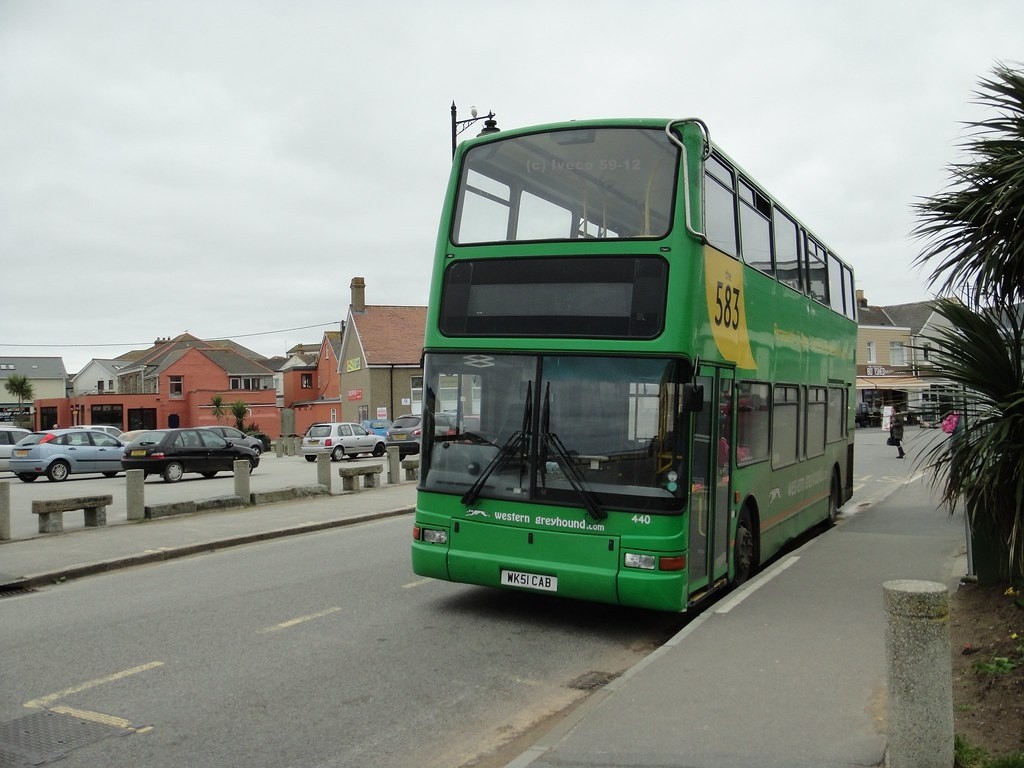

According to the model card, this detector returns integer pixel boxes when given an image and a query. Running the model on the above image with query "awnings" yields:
[856,376,931,393]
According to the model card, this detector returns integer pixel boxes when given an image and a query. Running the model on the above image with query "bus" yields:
[407,114,861,614]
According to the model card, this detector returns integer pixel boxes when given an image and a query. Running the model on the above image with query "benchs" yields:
[33,493,114,533]
[402,459,420,481]
[338,463,384,491]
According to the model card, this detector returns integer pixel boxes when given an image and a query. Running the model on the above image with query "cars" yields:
[57,425,125,445]
[360,419,394,439]
[301,422,387,462]
[10,428,127,483]
[120,428,261,483]
[0,427,34,472]
[177,426,265,456]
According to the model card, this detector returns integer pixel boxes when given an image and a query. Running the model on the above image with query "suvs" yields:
[448,415,481,436]
[386,412,457,462]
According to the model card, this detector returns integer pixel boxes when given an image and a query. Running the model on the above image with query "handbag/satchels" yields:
[887,437,896,446]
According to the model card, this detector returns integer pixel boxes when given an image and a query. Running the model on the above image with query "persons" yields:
[673,397,761,468]
[889,404,906,458]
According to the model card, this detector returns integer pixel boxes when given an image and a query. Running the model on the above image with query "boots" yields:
[896,447,905,458]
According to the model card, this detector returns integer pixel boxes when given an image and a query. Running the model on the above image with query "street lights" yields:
[447,98,504,435]
